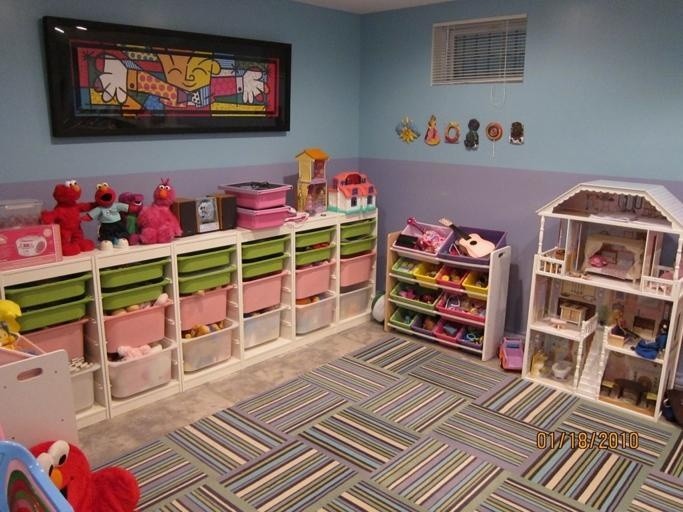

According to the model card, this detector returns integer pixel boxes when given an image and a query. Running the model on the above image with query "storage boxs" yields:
[389,222,507,349]
[216,180,294,230]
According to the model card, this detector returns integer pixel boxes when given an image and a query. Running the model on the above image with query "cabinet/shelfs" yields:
[1,207,379,431]
[385,231,512,362]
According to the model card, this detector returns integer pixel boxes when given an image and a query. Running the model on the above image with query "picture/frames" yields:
[40,15,292,137]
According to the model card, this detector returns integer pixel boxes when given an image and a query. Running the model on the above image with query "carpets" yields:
[89,334,682,511]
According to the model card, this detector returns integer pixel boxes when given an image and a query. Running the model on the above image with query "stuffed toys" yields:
[115,189,142,237]
[0,296,34,355]
[9,438,141,512]
[134,181,184,246]
[79,181,133,249]
[111,283,227,360]
